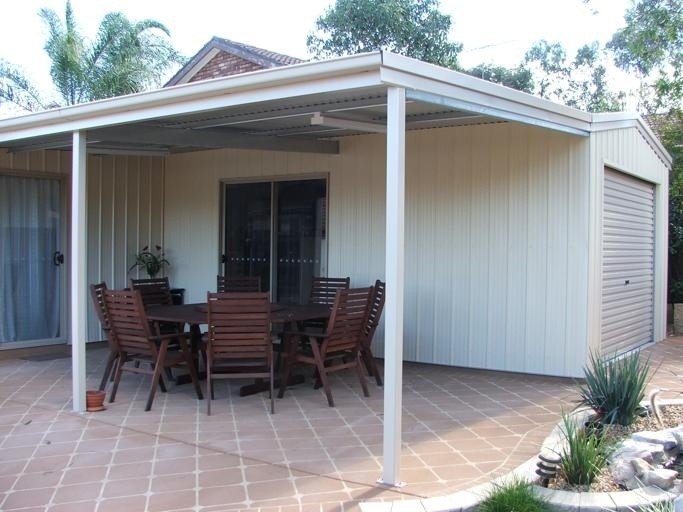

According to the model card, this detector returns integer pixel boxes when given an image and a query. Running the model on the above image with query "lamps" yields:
[310,114,387,135]
[535,450,563,488]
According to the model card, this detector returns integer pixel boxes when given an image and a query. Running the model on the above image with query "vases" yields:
[583,420,604,449]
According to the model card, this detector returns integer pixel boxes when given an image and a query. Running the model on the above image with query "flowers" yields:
[127,244,170,278]
